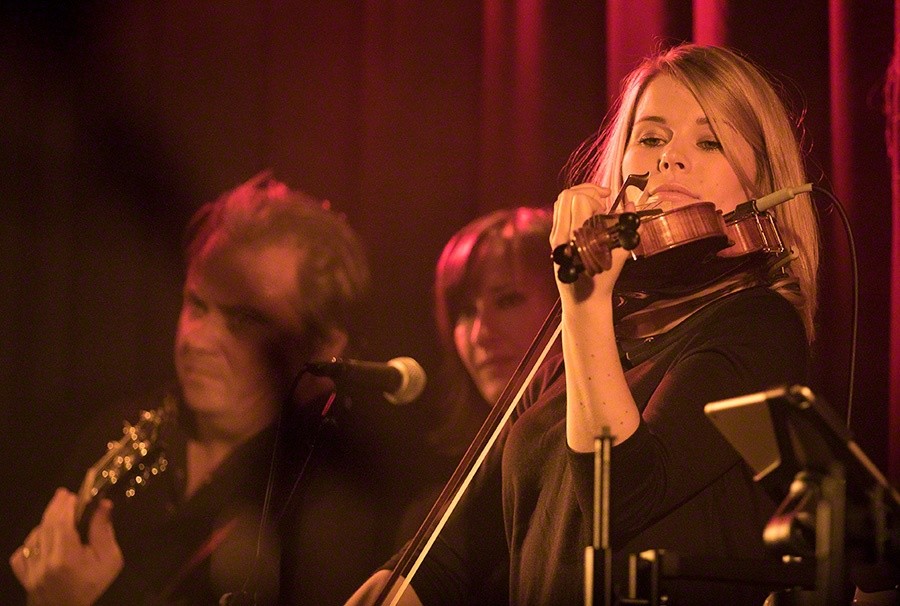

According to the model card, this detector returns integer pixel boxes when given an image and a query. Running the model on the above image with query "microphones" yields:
[306,357,427,405]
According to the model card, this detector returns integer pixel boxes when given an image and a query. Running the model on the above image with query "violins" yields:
[573,198,788,281]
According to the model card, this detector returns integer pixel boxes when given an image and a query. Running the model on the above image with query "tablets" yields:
[707,386,900,594]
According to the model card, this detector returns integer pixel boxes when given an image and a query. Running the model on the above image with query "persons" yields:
[8,43,818,606]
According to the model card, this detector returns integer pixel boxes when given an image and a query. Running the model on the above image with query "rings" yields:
[19,549,43,556]
